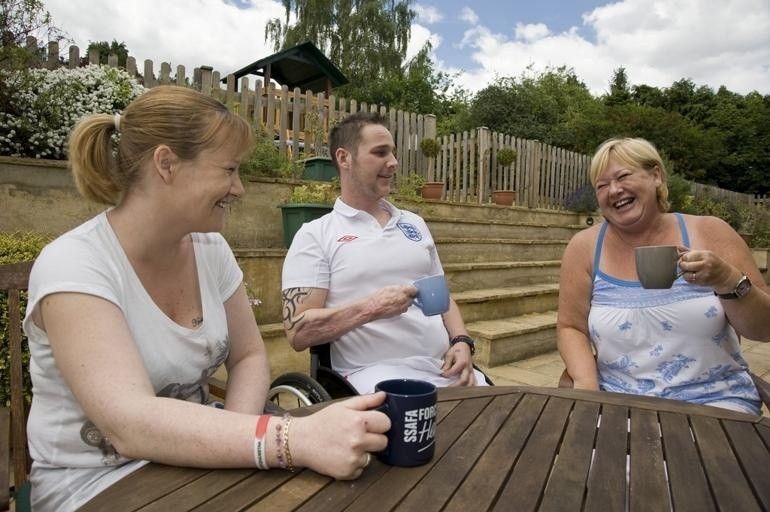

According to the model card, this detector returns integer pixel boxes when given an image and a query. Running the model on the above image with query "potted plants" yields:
[276,179,342,250]
[418,135,444,200]
[492,147,520,206]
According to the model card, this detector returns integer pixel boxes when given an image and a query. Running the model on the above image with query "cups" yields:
[368,378,438,467]
[412,273,450,317]
[635,246,688,288]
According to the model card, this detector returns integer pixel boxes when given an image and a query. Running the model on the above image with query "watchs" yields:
[713,274,752,300]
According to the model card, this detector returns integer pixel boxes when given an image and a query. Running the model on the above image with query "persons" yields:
[280,108,490,404]
[19,85,393,512]
[557,136,770,415]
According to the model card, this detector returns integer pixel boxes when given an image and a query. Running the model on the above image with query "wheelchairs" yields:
[267,344,495,407]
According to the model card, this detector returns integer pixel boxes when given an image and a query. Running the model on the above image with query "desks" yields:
[71,382,769,511]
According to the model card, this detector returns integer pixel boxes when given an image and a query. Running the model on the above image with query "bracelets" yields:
[284,415,293,473]
[274,420,287,470]
[451,333,475,354]
[256,413,270,471]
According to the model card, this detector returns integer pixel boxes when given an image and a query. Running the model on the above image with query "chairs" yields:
[1,261,285,506]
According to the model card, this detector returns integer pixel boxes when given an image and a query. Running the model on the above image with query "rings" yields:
[364,454,373,468]
[692,272,696,282]
